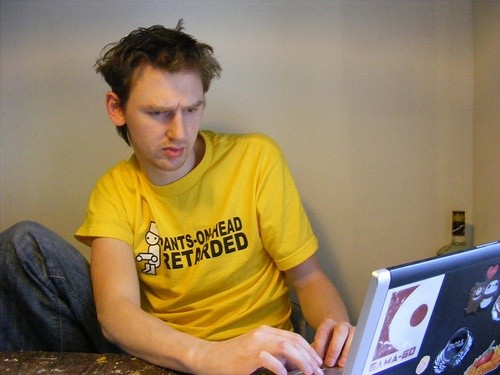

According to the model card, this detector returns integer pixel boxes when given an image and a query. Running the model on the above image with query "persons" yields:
[0,17,359,375]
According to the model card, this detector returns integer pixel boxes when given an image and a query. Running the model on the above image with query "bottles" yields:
[436,209,475,260]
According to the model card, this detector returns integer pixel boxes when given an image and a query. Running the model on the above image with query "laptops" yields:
[272,240,499,375]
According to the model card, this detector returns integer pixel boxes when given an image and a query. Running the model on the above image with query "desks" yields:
[0,350,192,375]
